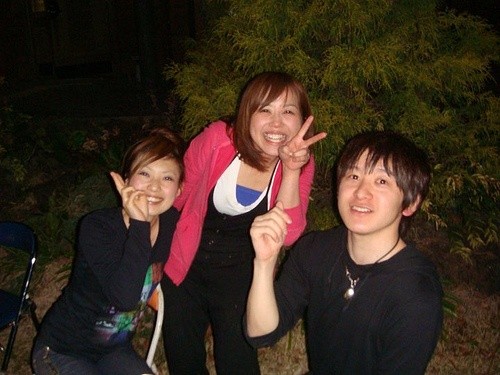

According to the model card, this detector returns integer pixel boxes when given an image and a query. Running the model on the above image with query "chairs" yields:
[0,220,42,374]
[145,281,165,375]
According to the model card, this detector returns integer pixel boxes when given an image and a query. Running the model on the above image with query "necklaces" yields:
[341,234,401,303]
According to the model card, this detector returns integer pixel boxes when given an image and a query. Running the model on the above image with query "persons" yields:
[242,130,446,375]
[160,71,328,375]
[28,127,188,375]
